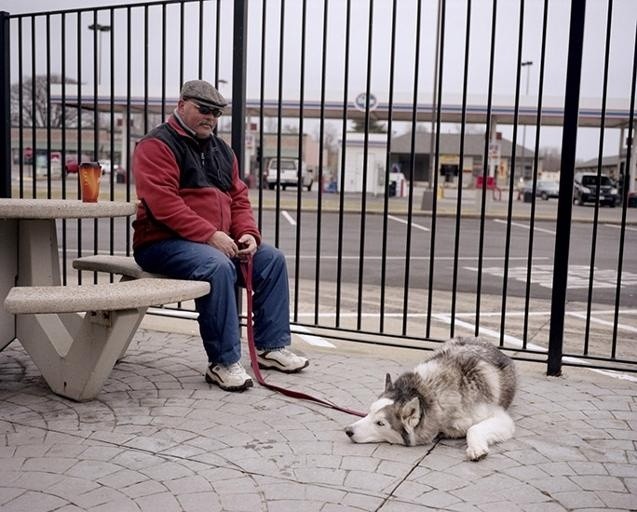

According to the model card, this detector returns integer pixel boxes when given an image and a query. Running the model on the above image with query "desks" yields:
[1,196,140,403]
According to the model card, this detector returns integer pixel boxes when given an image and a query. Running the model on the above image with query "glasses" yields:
[189,101,223,117]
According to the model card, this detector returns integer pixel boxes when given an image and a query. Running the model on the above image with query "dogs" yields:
[345,334,518,463]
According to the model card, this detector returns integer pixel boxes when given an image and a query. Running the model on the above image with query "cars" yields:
[573,171,621,208]
[65,154,111,174]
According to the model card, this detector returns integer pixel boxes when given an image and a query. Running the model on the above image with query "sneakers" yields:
[205,362,254,392]
[251,346,309,373]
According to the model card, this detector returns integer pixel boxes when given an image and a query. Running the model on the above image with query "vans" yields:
[266,157,315,191]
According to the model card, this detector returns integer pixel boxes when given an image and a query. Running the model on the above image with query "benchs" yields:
[2,254,212,404]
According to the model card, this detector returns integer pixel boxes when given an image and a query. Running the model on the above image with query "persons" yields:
[128,78,311,394]
[607,169,622,189]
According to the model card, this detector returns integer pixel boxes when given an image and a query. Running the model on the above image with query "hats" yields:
[181,80,227,110]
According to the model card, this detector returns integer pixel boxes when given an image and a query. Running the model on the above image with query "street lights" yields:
[521,61,533,157]
[88,23,110,86]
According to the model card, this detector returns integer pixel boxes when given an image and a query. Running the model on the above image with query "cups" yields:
[76,159,103,203]
